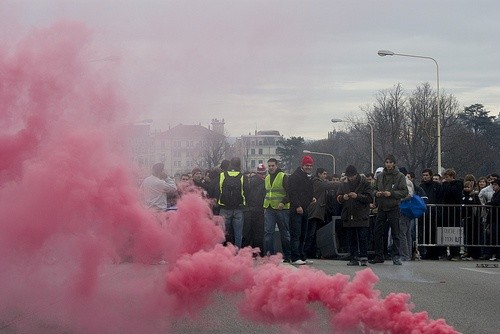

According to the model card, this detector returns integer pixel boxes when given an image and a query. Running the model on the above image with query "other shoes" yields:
[304,259,315,263]
[347,260,359,266]
[292,259,306,265]
[392,257,402,265]
[368,258,383,263]
[420,255,500,261]
[361,260,367,266]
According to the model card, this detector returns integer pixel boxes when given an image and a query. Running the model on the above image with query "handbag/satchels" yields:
[400,194,426,219]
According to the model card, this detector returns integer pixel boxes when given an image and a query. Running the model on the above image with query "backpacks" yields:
[220,172,244,205]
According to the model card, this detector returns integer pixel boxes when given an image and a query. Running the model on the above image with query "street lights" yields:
[332,120,374,175]
[304,150,336,174]
[377,49,443,175]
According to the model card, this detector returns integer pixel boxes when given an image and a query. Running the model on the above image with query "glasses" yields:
[268,165,275,167]
[182,178,188,180]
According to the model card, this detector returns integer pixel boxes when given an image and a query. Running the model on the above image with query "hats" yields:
[256,164,266,173]
[302,156,313,166]
[192,168,201,177]
[345,165,357,176]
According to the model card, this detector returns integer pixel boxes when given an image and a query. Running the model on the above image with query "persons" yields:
[138,155,500,267]
